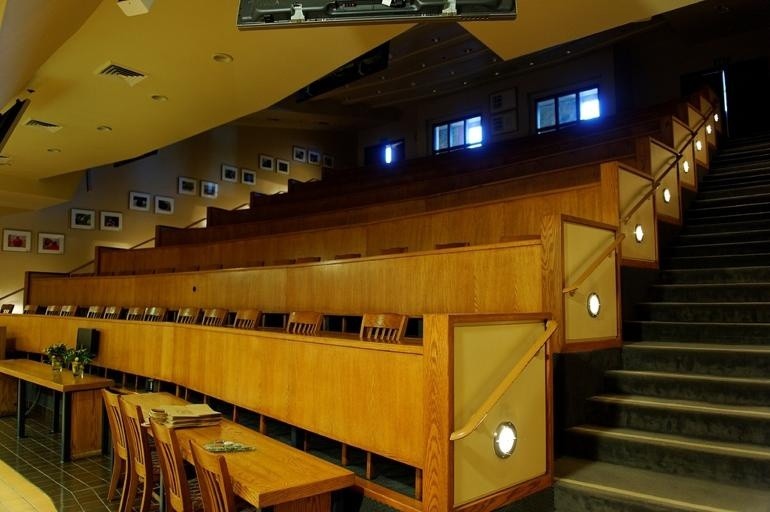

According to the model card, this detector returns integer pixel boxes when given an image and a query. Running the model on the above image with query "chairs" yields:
[1,304,409,510]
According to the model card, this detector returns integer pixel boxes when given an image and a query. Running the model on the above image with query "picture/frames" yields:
[1,228,32,251]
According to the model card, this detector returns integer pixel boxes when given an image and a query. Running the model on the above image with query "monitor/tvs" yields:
[1,97,32,158]
[234,0,519,33]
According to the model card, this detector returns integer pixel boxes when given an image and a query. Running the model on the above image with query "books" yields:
[155,403,224,430]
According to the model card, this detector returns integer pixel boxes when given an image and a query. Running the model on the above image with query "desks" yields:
[23,85,724,353]
